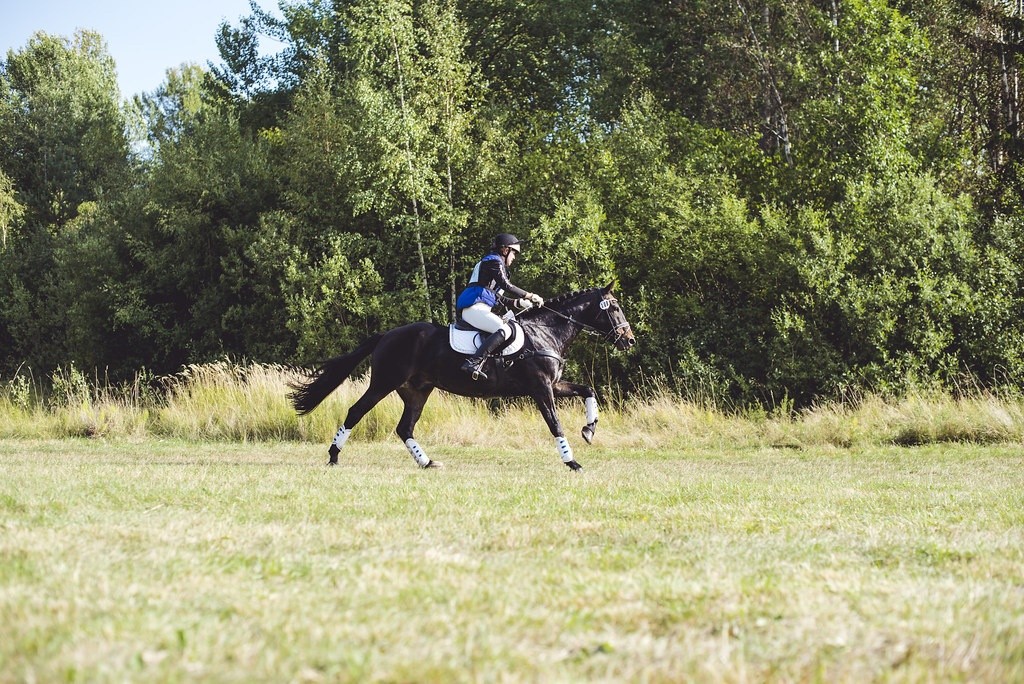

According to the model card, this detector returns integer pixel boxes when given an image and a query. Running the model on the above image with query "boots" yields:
[460,329,506,382]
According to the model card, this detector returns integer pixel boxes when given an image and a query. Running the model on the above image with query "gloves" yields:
[525,292,544,308]
[514,298,533,308]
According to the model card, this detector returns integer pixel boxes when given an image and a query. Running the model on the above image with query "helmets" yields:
[491,234,524,253]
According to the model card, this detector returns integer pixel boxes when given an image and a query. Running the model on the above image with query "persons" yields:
[456,233,544,381]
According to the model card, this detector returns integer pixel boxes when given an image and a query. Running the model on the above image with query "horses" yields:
[284,279,635,472]
